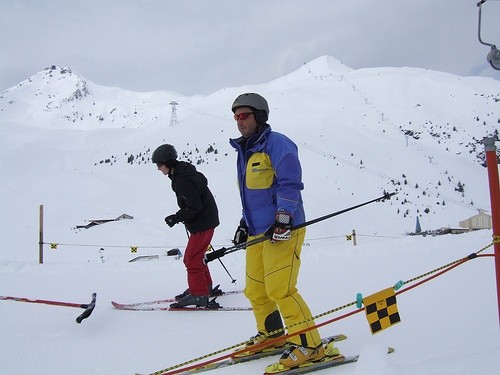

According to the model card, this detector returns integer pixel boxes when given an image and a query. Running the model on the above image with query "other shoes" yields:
[279,341,326,367]
[246,332,286,352]
[178,294,209,308]
[175,290,190,300]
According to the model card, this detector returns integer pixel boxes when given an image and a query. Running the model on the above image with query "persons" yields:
[228,92,326,369]
[149,142,220,310]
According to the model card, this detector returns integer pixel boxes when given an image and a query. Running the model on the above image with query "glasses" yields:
[234,112,254,120]
[157,161,164,170]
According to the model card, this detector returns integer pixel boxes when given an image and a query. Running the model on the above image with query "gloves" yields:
[165,214,178,228]
[233,225,247,249]
[270,212,292,244]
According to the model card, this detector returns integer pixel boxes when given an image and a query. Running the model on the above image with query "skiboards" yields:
[112,289,252,311]
[133,334,359,374]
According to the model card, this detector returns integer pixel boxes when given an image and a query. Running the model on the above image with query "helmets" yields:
[232,93,269,121]
[152,144,177,163]
